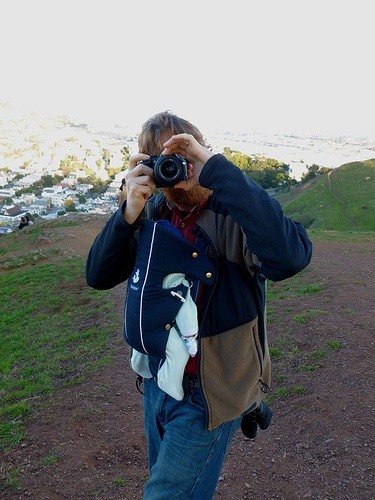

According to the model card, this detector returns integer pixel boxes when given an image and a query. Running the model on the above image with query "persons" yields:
[18,213,34,230]
[85,109,312,500]
[130,219,199,401]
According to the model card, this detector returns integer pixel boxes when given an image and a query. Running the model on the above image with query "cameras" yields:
[141,156,187,188]
